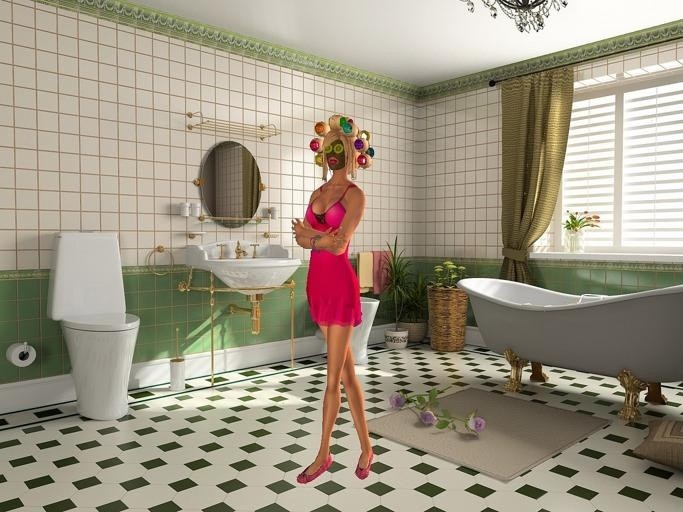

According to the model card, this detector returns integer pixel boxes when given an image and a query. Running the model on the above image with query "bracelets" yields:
[308,237,317,250]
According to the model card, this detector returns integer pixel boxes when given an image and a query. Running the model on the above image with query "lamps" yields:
[459,0,568,34]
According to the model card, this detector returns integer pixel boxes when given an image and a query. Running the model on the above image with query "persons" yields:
[288,112,375,484]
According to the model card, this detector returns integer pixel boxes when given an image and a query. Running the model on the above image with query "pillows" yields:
[633,419,682,473]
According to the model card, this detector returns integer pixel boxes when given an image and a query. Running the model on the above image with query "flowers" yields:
[560,209,602,232]
[389,384,486,435]
[429,261,467,289]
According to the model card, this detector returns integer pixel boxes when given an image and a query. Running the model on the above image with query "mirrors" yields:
[194,141,266,229]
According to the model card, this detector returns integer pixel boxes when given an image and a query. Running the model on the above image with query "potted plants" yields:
[379,234,430,350]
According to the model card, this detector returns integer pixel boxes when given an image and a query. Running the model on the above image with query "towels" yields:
[355,250,392,294]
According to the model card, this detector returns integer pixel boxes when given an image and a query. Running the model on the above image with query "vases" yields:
[427,285,468,352]
[569,232,581,253]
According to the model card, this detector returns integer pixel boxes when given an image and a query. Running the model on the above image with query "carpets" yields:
[352,388,614,482]
